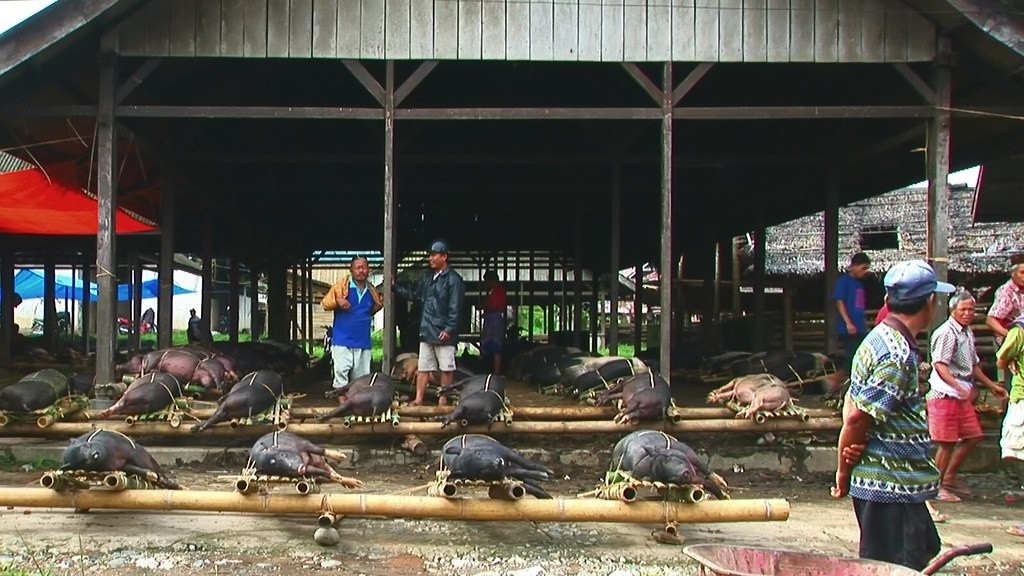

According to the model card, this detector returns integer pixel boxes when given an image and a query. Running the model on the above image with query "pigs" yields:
[0,328,859,503]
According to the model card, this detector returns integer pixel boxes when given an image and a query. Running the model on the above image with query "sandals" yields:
[932,510,947,523]
[1006,526,1024,536]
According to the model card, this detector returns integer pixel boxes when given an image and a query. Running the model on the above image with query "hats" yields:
[1008,254,1024,267]
[429,242,447,254]
[884,260,955,300]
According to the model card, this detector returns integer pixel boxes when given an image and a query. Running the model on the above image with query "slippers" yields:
[936,492,962,503]
[401,400,417,407]
[945,482,974,495]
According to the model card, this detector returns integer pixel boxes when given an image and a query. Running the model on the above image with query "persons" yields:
[142,307,155,334]
[831,260,956,571]
[390,241,464,406]
[824,252,1024,538]
[474,270,507,375]
[189,309,228,342]
[322,256,384,405]
[14,291,22,335]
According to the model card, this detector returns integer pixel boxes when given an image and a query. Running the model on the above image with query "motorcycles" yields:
[30,311,71,334]
[116,308,158,336]
